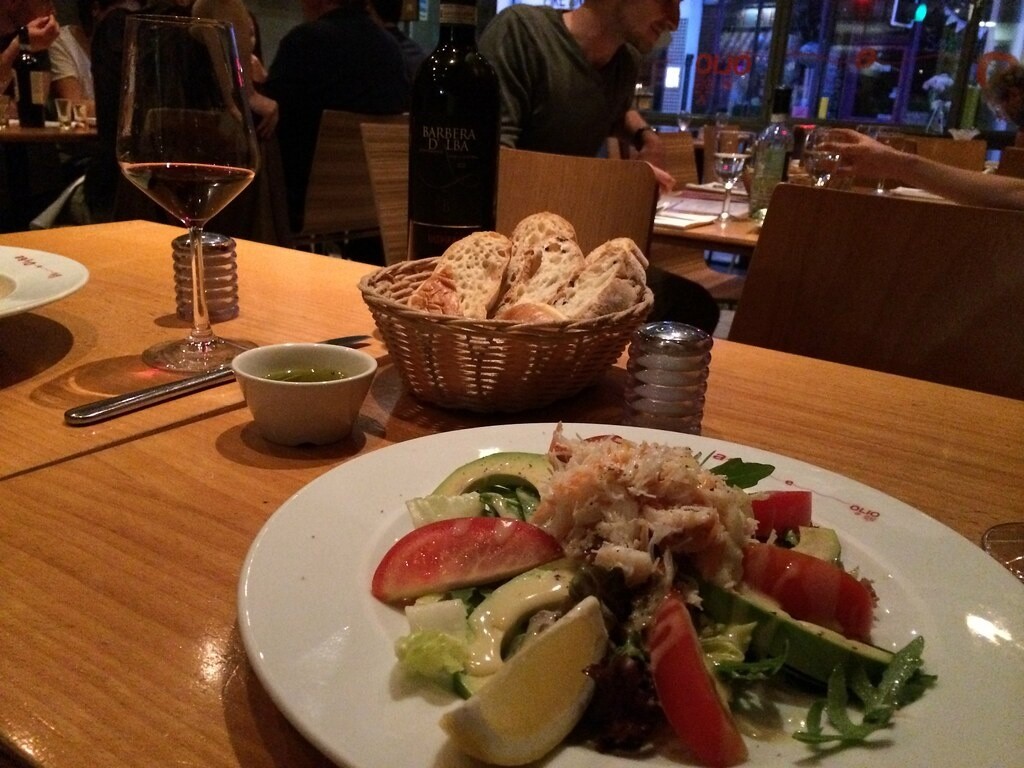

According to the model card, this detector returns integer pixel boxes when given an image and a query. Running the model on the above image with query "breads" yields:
[402,208,648,397]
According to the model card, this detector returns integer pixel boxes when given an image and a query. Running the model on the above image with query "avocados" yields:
[700,579,899,689]
[779,525,841,566]
[451,555,567,701]
[428,451,555,498]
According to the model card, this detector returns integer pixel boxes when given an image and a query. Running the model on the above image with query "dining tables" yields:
[648,167,953,251]
[0,120,99,144]
[0,219,400,485]
[0,335,1024,768]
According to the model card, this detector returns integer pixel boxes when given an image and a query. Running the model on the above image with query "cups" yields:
[800,130,840,186]
[54,98,72,133]
[677,111,691,131]
[73,103,88,131]
[716,112,729,128]
[748,141,784,227]
[233,343,378,446]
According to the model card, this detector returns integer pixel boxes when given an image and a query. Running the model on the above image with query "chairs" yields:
[297,111,1024,404]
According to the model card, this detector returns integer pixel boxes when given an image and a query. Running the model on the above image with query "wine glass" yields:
[116,14,259,374]
[711,129,756,230]
[983,522,1024,586]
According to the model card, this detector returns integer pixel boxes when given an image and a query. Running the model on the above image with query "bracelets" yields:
[634,125,658,152]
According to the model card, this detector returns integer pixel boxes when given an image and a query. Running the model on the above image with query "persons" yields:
[0,0,426,248]
[810,50,1024,212]
[474,0,721,338]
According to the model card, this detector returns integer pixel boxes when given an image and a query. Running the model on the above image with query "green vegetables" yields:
[399,456,937,745]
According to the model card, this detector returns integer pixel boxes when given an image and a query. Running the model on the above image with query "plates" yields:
[653,211,718,229]
[238,423,1024,768]
[0,245,89,317]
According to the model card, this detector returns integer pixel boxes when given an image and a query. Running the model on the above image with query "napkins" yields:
[891,186,947,200]
[651,210,721,230]
[685,181,749,198]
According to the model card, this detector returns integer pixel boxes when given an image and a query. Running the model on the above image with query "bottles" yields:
[172,233,239,322]
[621,322,713,436]
[759,113,793,183]
[11,27,45,129]
[407,0,502,263]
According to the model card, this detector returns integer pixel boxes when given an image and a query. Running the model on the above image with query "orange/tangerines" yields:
[440,596,607,766]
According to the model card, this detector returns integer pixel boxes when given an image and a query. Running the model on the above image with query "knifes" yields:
[63,336,372,425]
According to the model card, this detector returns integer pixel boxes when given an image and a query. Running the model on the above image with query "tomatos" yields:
[647,595,749,766]
[735,540,875,644]
[739,490,816,538]
[373,516,568,600]
